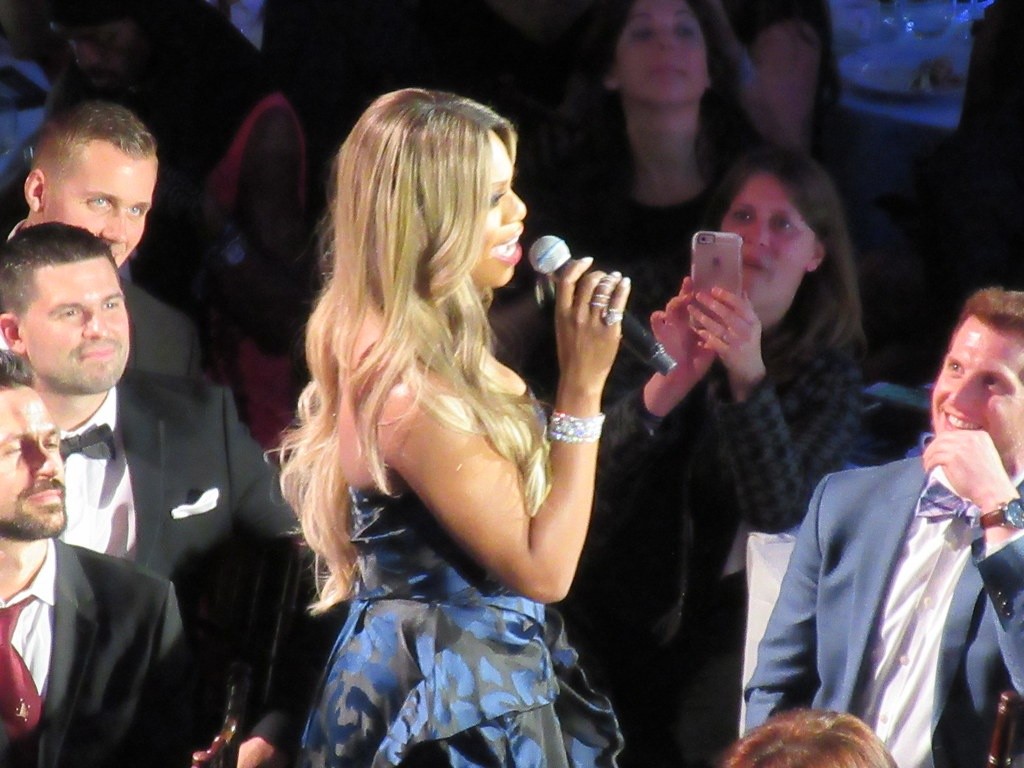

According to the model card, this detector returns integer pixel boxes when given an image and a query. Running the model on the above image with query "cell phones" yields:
[690,231,743,330]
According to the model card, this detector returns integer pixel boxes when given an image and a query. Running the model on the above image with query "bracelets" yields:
[547,408,606,444]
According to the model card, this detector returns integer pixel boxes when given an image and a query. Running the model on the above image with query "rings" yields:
[591,302,609,307]
[593,294,611,299]
[605,308,624,325]
[598,275,618,290]
[721,333,728,342]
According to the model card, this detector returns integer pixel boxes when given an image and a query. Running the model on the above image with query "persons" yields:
[743,284,1024,768]
[0,221,351,768]
[43,1,321,464]
[0,100,204,379]
[592,145,869,768]
[709,706,900,768]
[260,0,1024,249]
[570,1,770,271]
[263,86,626,768]
[0,350,197,768]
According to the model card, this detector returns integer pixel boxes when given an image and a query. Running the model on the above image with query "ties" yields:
[1,594,44,747]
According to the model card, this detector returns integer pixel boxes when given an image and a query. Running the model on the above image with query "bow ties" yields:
[59,423,116,463]
[915,477,981,530]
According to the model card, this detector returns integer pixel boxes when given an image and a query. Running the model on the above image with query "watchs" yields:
[976,497,1024,530]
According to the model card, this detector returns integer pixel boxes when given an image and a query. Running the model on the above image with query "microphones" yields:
[529,235,678,377]
